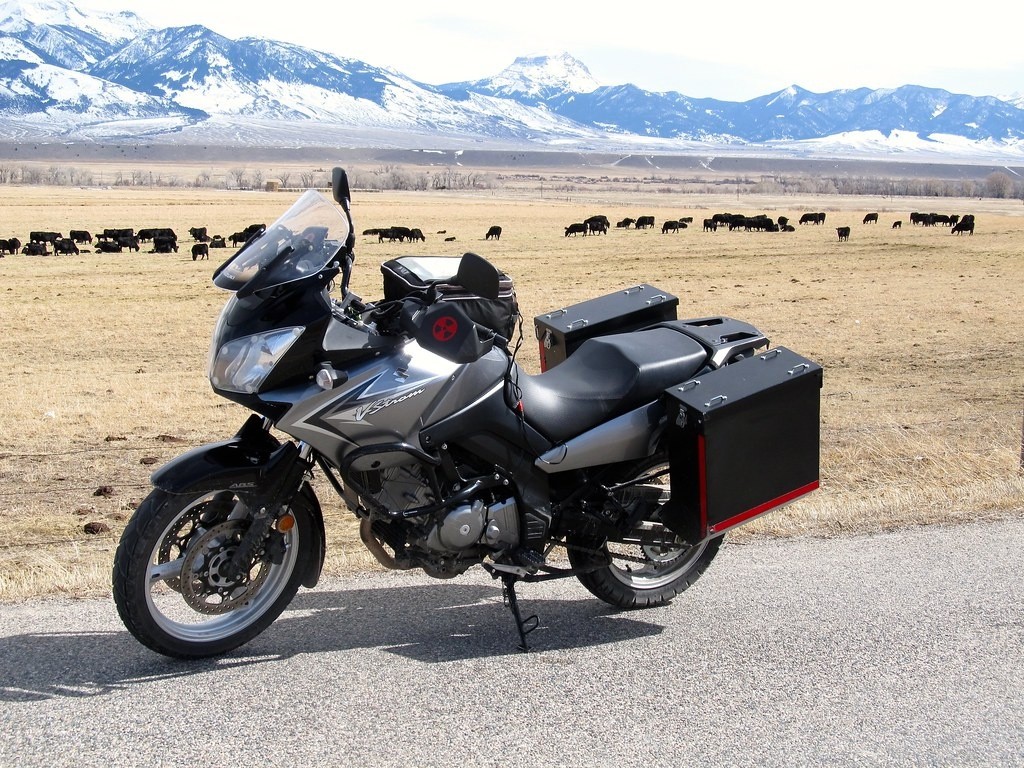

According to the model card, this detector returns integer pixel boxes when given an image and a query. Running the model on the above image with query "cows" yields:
[565,213,795,237]
[363,226,502,243]
[893,221,901,228]
[0,224,266,261]
[836,227,850,242]
[910,212,974,236]
[799,213,826,225]
[863,213,878,224]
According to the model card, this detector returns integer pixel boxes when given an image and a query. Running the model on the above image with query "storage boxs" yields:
[534,283,679,374]
[666,345,823,547]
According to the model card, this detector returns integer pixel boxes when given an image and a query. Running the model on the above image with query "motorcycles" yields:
[109,167,774,660]
